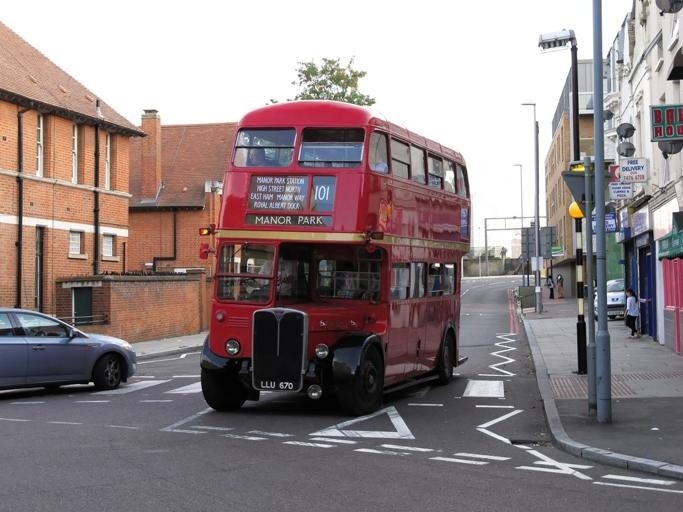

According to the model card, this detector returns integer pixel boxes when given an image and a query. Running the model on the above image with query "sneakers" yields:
[630,330,640,339]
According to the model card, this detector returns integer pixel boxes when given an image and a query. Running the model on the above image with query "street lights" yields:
[538,26,592,374]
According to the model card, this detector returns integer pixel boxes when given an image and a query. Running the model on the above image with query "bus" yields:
[197,99,471,410]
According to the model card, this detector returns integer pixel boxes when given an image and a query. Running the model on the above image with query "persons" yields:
[622,288,639,338]
[371,142,388,173]
[547,275,555,299]
[249,146,281,167]
[255,251,280,286]
[555,272,564,299]
[276,259,305,296]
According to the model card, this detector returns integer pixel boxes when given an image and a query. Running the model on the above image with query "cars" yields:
[593,276,627,320]
[0,307,138,395]
